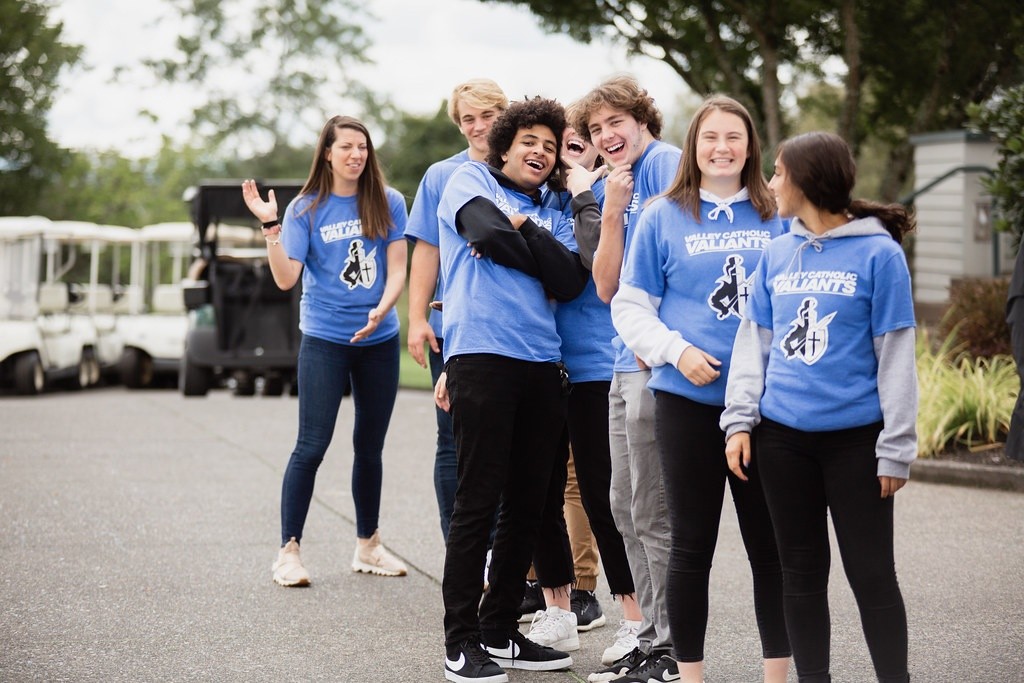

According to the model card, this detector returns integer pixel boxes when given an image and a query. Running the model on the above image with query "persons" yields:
[403,77,683,683]
[610,94,792,683]
[242,115,409,586]
[719,131,919,683]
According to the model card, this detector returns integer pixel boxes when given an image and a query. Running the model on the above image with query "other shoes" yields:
[483,549,492,590]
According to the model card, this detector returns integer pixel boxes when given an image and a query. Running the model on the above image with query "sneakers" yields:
[271,536,311,586]
[352,529,408,576]
[587,647,680,683]
[445,639,508,683]
[570,589,606,631]
[517,579,547,622]
[524,606,580,651]
[602,619,642,666]
[480,629,572,671]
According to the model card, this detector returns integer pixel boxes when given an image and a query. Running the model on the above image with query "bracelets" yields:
[261,217,282,246]
